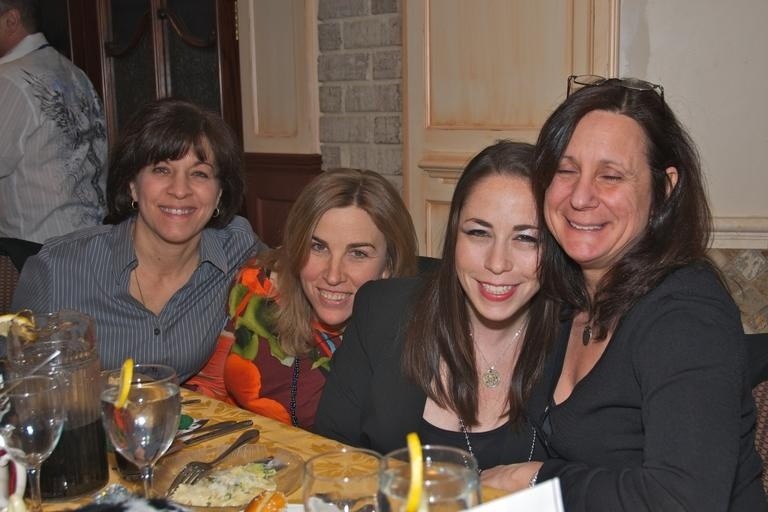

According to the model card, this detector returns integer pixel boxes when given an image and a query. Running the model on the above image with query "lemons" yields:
[114,357,134,409]
[404,432,425,512]
[0,314,36,342]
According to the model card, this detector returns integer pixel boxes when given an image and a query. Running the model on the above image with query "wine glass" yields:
[100,364,182,499]
[0,374,67,511]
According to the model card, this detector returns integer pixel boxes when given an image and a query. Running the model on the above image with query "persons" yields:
[313,139,550,475]
[0,1,113,275]
[468,70,766,511]
[180,165,420,438]
[11,96,275,383]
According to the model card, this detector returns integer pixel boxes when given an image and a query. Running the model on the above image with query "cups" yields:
[6,308,110,504]
[302,444,483,512]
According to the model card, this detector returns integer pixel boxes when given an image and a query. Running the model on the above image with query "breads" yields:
[245,489,289,512]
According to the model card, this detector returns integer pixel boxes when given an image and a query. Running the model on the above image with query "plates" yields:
[152,442,306,512]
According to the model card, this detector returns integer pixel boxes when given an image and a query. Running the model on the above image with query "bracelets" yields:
[525,470,541,490]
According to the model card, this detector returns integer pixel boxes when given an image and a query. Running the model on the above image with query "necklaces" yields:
[453,415,537,470]
[468,317,529,390]
[580,314,599,348]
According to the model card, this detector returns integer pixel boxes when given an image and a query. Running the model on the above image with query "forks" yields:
[168,429,261,496]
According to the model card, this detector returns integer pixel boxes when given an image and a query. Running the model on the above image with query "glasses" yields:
[566,75,666,115]
